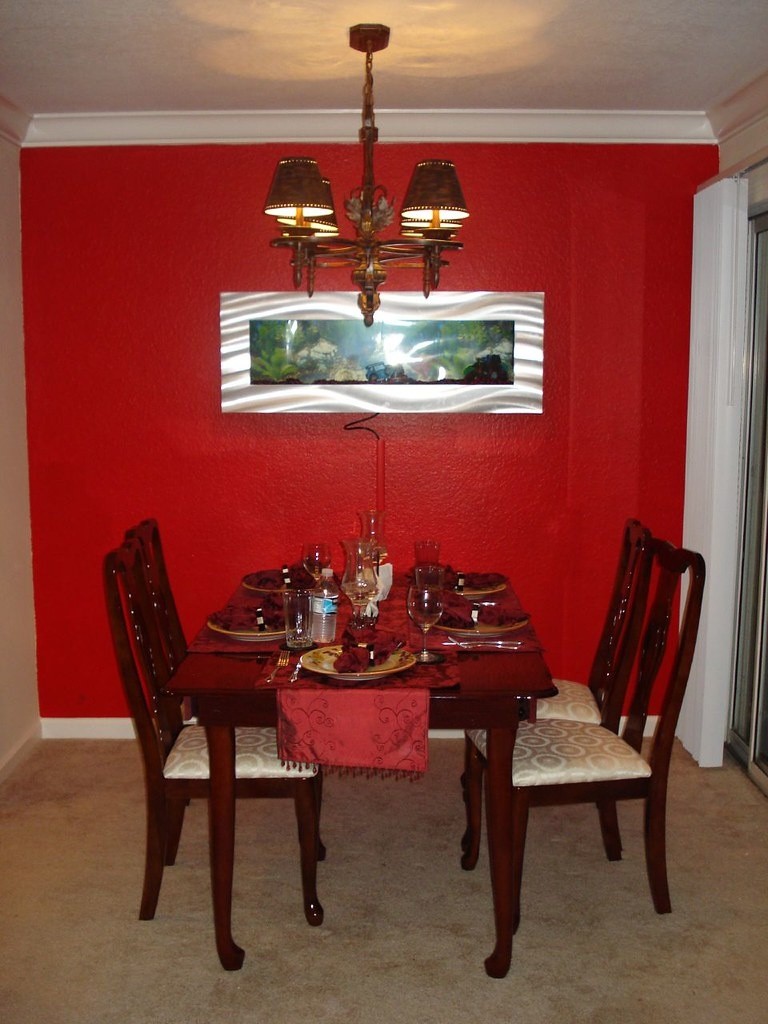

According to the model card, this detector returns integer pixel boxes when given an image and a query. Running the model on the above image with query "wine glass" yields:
[341,538,378,632]
[407,585,444,665]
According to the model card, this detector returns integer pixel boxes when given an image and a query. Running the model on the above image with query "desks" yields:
[165,572,559,979]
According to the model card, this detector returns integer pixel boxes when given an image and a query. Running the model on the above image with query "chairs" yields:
[105,518,326,926]
[462,518,705,934]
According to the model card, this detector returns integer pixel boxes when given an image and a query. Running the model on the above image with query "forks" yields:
[447,635,518,650]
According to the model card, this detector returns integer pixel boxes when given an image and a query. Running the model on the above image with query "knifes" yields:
[442,642,523,646]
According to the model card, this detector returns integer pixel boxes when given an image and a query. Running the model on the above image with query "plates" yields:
[442,580,505,601]
[301,645,415,681]
[207,613,287,643]
[434,608,530,638]
[240,570,314,594]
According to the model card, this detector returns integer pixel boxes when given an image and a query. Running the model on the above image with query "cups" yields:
[413,539,439,568]
[281,590,313,648]
[414,563,442,587]
[303,544,331,586]
[355,508,387,571]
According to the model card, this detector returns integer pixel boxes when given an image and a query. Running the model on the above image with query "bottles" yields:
[309,568,339,643]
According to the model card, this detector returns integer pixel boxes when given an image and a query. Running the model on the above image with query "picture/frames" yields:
[221,291,544,415]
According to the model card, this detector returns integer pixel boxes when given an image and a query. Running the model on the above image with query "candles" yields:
[377,438,385,513]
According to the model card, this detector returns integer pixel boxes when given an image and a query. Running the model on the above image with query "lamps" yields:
[263,24,470,328]
